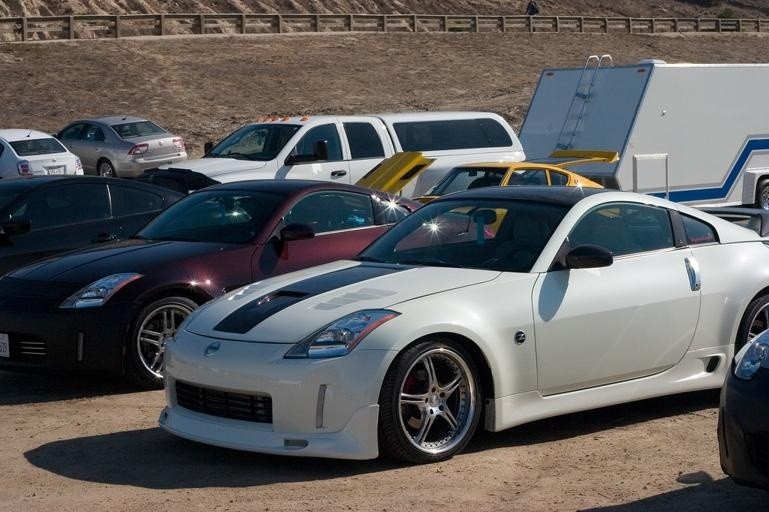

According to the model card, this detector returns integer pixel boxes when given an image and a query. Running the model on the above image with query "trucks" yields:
[519,56,769,210]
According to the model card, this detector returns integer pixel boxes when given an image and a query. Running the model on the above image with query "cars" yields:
[52,117,188,177]
[0,176,185,264]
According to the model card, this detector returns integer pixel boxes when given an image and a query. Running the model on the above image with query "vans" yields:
[0,129,85,177]
[142,112,526,199]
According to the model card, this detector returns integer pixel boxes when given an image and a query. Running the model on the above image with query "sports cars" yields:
[716,326,769,488]
[355,150,620,238]
[160,186,769,465]
[0,178,497,388]
